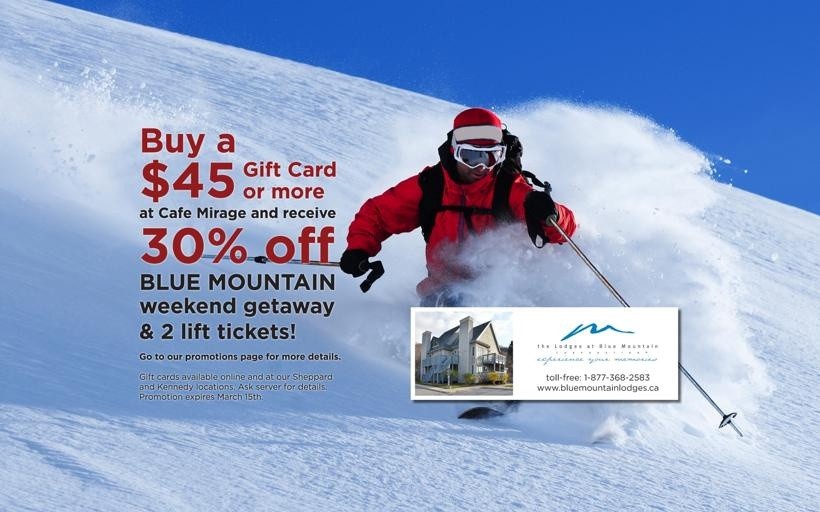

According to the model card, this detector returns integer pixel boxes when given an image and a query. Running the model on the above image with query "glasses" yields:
[453,143,508,169]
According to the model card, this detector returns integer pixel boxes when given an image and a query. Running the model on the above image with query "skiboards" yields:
[456,402,519,418]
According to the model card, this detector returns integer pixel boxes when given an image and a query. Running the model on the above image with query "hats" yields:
[454,109,504,146]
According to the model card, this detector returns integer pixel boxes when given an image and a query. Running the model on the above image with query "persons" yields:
[340,109,579,418]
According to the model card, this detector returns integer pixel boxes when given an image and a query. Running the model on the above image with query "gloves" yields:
[340,248,368,277]
[522,189,558,226]
